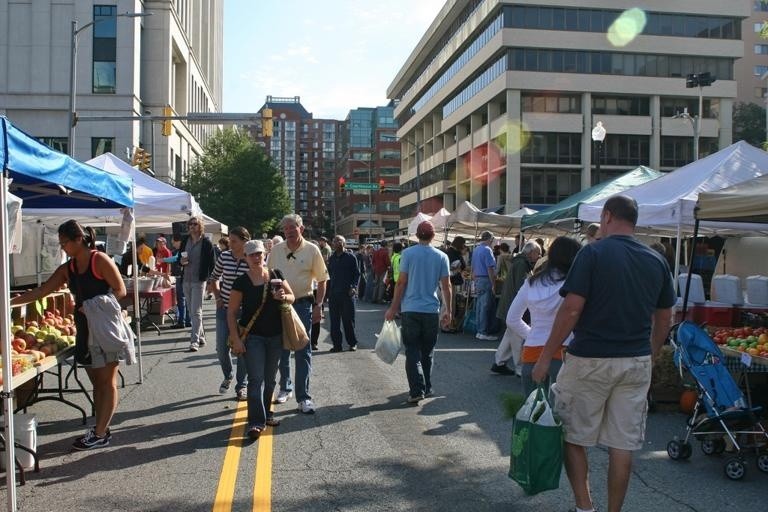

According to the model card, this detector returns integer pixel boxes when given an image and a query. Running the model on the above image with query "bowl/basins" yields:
[123,275,162,292]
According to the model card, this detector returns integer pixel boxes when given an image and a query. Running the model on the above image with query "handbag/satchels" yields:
[280,303,310,350]
[509,414,564,495]
[227,324,248,349]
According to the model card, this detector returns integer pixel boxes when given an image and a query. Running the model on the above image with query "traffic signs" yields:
[346,182,379,190]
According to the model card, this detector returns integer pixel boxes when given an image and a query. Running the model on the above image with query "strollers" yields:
[666,321,768,480]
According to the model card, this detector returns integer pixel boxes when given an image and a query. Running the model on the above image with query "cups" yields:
[271,279,282,295]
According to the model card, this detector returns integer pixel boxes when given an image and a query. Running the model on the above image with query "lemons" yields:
[748,333,768,355]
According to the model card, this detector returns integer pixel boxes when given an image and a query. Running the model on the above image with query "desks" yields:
[457,291,501,332]
[122,284,178,336]
[0,316,132,484]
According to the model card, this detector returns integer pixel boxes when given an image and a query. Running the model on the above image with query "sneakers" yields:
[407,395,425,402]
[275,390,292,403]
[219,376,234,392]
[476,332,499,341]
[491,363,514,375]
[248,427,260,437]
[191,342,199,351]
[266,418,279,425]
[298,400,315,414]
[330,348,342,352]
[200,336,206,345]
[238,387,247,400]
[72,426,112,450]
[350,345,357,351]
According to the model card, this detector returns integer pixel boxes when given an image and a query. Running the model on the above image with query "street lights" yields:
[383,134,422,214]
[348,158,371,235]
[66,9,155,158]
[671,111,699,163]
[591,121,606,184]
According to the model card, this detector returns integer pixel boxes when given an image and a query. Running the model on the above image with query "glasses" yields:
[189,222,198,225]
[59,239,70,246]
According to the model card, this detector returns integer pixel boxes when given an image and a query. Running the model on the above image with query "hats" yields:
[588,223,602,239]
[243,240,265,256]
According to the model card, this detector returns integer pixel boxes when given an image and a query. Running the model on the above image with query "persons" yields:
[385,220,452,402]
[227,240,296,435]
[532,195,678,512]
[11,221,127,453]
[123,213,725,435]
[209,227,252,399]
[266,214,331,414]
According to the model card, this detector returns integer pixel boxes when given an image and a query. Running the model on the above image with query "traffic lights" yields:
[339,178,345,193]
[380,181,385,192]
[131,147,144,168]
[140,152,152,170]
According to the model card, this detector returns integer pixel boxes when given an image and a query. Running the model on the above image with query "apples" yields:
[713,326,768,348]
[11,309,76,362]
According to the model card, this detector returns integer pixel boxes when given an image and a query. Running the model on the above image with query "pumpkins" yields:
[680,390,698,412]
